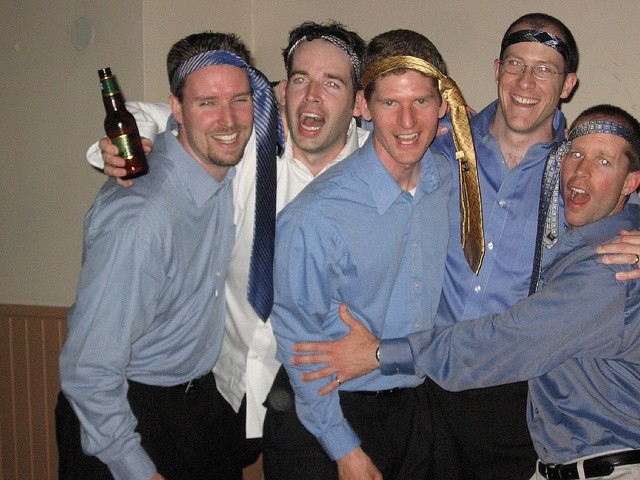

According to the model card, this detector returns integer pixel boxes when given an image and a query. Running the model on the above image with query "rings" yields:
[631,254,639,266]
[336,377,341,385]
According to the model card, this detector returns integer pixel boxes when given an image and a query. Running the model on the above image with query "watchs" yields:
[376,347,380,361]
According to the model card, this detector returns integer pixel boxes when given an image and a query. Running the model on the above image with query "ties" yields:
[361,56,487,274]
[285,32,361,85]
[528,118,638,297]
[172,49,285,323]
[502,28,570,77]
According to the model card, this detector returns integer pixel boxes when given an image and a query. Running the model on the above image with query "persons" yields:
[261,27,454,480]
[428,12,640,480]
[53,29,252,480]
[86,18,449,480]
[291,102,640,480]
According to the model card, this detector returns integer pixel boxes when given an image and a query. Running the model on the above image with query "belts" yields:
[536,451,640,480]
